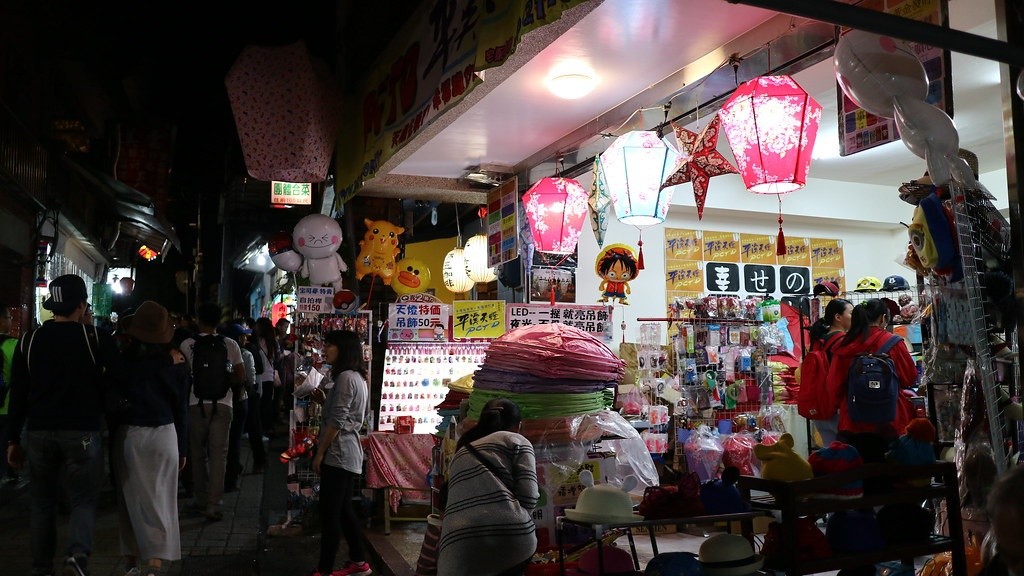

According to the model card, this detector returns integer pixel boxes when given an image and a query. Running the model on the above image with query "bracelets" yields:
[7,440,19,445]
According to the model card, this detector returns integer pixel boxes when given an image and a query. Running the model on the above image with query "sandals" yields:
[200,509,224,521]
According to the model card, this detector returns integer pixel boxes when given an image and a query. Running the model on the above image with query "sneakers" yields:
[64,557,85,576]
[329,560,372,576]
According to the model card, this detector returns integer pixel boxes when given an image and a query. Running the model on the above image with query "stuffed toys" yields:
[905,202,958,277]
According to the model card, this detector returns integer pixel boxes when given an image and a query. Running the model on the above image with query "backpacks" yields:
[798,332,848,420]
[191,334,230,400]
[847,335,904,422]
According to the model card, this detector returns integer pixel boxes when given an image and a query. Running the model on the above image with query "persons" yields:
[792,298,917,452]
[436,398,541,576]
[307,329,372,576]
[0,303,295,520]
[104,302,193,576]
[9,273,116,576]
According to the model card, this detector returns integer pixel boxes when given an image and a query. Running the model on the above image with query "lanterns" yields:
[224,29,335,184]
[598,131,678,275]
[715,72,822,257]
[523,174,588,309]
[442,231,499,302]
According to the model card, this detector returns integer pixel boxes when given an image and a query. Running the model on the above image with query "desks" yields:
[368,430,437,538]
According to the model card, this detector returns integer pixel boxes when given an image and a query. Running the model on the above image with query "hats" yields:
[639,486,681,514]
[754,418,936,559]
[121,301,174,343]
[854,276,883,291]
[228,324,252,334]
[879,275,910,291]
[700,482,752,514]
[646,552,704,576]
[42,274,88,310]
[564,470,645,522]
[700,534,764,576]
[575,546,642,576]
[814,282,839,296]
